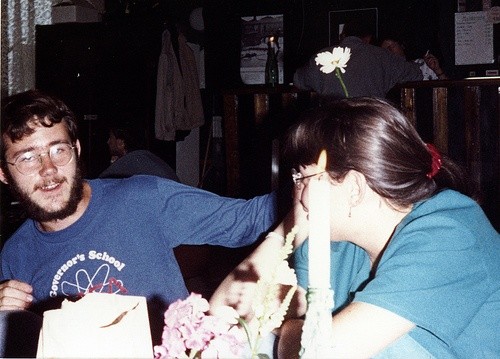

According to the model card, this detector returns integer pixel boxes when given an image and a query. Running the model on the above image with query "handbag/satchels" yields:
[30,291,155,357]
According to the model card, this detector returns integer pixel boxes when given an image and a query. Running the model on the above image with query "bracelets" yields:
[266,232,297,252]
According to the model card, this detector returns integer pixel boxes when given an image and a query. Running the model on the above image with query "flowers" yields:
[152,223,300,359]
[315,46,354,98]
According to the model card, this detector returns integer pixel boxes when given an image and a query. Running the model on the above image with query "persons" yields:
[0,90,297,359]
[292,15,422,110]
[207,96,500,359]
[379,37,408,62]
[95,118,177,182]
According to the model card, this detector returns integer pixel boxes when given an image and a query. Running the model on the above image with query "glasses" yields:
[289,166,331,189]
[2,144,79,175]
